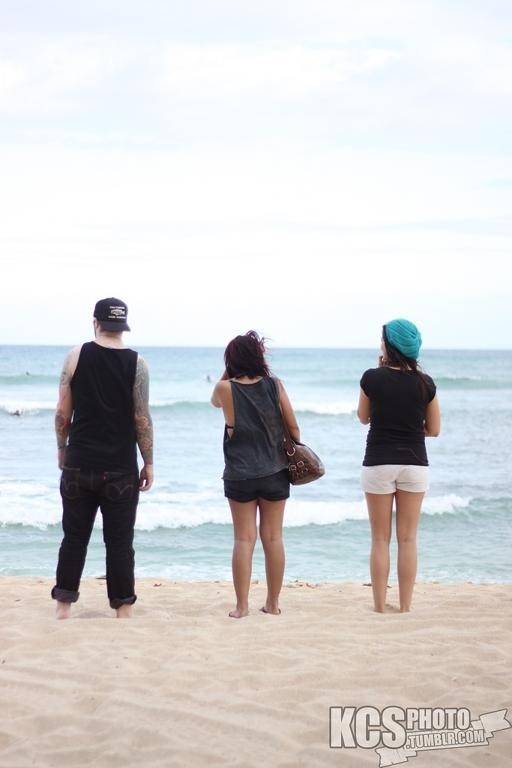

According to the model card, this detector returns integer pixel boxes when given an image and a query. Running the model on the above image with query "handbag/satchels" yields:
[284,440,325,485]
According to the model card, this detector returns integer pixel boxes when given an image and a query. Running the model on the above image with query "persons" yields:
[357,316,440,613]
[50,297,153,620]
[211,327,301,618]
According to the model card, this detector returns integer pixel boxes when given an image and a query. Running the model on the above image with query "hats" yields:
[94,297,131,332]
[385,319,422,361]
